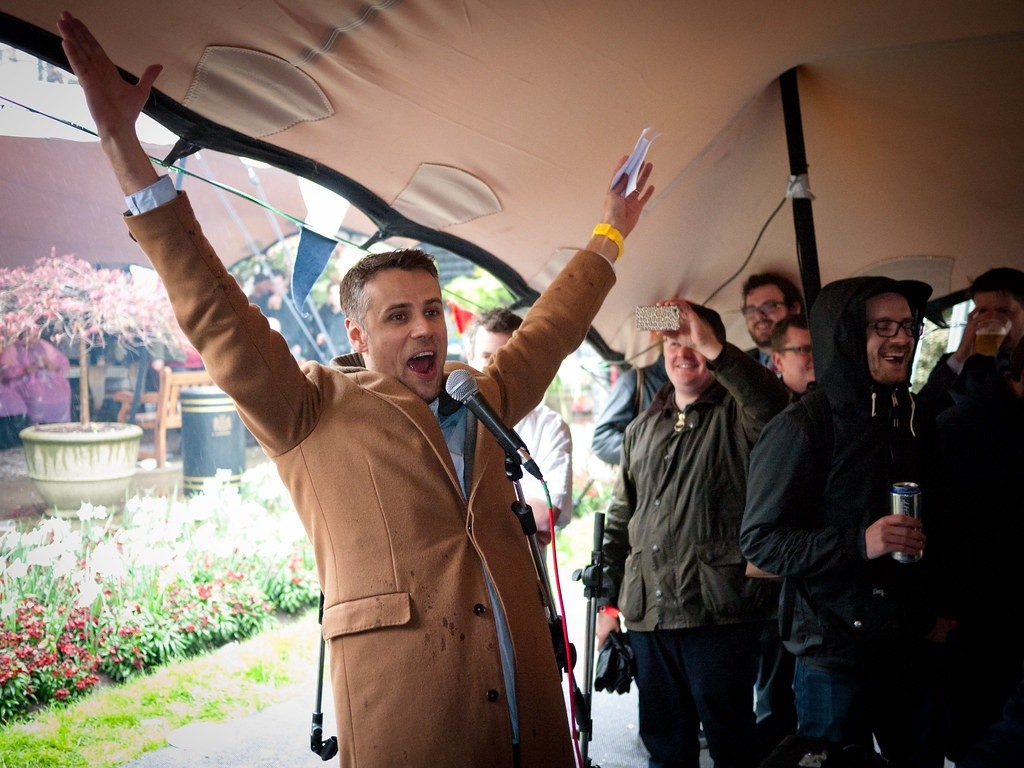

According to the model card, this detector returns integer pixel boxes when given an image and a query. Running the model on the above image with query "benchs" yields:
[113,369,215,470]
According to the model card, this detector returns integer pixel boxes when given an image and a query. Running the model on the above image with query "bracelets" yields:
[598,605,619,618]
[592,223,625,260]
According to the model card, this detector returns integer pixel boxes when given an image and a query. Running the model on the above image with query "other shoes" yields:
[698,728,709,750]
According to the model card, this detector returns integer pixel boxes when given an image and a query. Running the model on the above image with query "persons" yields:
[151,304,205,372]
[739,267,1024,768]
[741,272,805,370]
[465,308,573,551]
[769,313,816,402]
[596,298,791,768]
[0,322,71,451]
[590,351,669,466]
[57,11,655,768]
[249,269,351,365]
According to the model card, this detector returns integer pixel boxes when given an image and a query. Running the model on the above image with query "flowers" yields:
[0,246,195,429]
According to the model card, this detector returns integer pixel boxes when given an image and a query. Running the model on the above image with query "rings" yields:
[635,190,640,193]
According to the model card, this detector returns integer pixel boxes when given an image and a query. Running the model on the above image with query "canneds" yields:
[889,481,925,564]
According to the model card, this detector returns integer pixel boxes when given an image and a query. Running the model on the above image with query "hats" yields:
[864,280,934,312]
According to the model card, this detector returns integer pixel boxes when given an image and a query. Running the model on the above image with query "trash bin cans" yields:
[179,386,246,496]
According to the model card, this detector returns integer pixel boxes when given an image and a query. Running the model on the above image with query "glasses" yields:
[868,321,925,338]
[778,344,813,357]
[742,300,791,320]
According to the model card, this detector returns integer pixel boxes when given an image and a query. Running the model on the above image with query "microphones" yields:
[446,370,543,479]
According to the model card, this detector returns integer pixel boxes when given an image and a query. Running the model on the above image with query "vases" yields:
[18,421,143,518]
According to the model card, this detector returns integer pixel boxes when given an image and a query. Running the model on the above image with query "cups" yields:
[973,312,1012,356]
